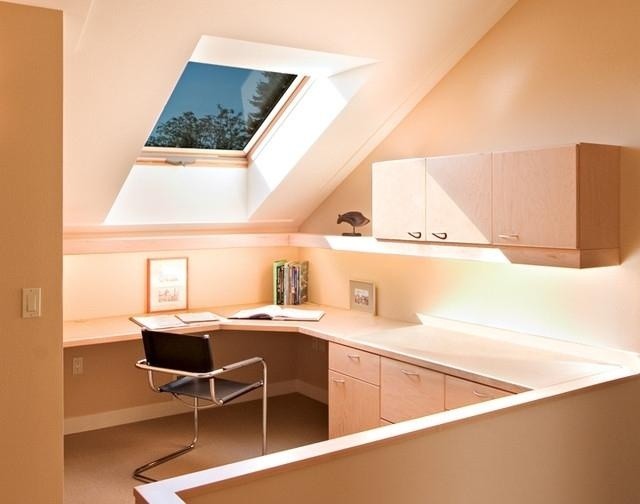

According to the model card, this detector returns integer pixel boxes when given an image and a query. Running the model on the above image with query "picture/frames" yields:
[347,278,376,316]
[144,255,191,314]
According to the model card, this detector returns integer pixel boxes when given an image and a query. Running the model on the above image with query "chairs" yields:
[131,325,269,487]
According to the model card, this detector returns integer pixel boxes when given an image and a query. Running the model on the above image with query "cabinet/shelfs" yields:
[322,339,381,441]
[366,152,491,248]
[133,356,640,501]
[486,138,623,269]
[444,366,518,412]
[378,351,444,426]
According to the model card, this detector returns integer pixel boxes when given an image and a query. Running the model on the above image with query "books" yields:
[228,305,325,323]
[274,258,310,306]
[129,310,222,329]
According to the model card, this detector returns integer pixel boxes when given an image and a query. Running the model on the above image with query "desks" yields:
[64,301,409,355]
